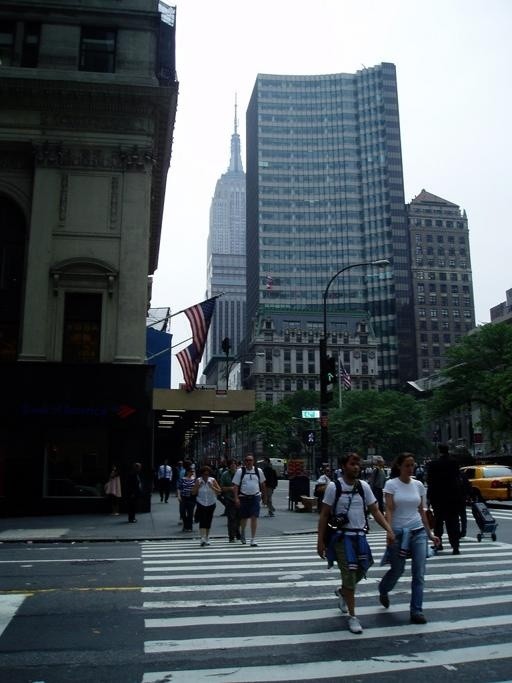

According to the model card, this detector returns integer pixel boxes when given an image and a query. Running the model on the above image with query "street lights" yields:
[321,257,391,476]
[228,351,267,381]
[291,415,316,474]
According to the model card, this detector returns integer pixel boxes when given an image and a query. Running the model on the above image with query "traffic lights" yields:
[432,430,439,441]
[307,430,315,445]
[325,354,336,386]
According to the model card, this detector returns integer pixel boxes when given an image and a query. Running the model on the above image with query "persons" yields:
[379,452,443,624]
[317,453,396,633]
[103,441,468,554]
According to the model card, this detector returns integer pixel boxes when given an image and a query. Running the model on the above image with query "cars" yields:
[195,454,428,485]
[471,442,489,457]
[458,462,512,504]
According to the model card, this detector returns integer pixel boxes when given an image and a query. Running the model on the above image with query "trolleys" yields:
[463,494,499,542]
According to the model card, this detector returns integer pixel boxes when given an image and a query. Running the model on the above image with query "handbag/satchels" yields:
[313,485,327,497]
[426,509,435,529]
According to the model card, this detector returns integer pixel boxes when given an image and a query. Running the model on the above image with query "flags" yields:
[340,360,351,391]
[266,274,272,292]
[174,344,200,393]
[182,296,216,358]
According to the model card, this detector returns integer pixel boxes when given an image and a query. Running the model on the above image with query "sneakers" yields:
[345,615,362,633]
[184,527,257,546]
[411,613,426,623]
[335,587,348,614]
[379,590,389,608]
